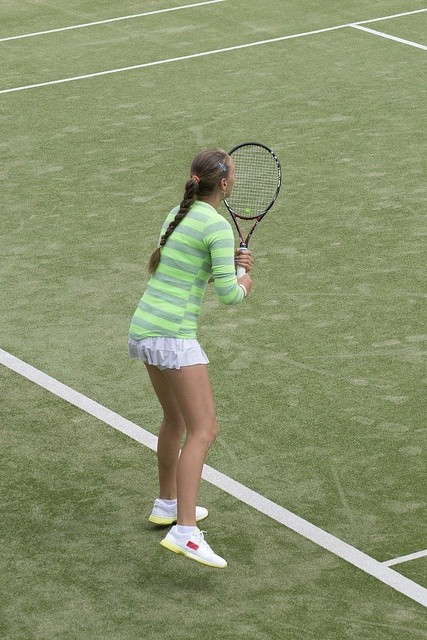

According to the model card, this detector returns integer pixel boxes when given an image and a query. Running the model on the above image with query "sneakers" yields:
[161,527,226,569]
[148,499,209,526]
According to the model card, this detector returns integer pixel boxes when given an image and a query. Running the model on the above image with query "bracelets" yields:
[235,282,249,300]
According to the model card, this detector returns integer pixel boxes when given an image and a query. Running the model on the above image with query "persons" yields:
[127,148,256,570]
